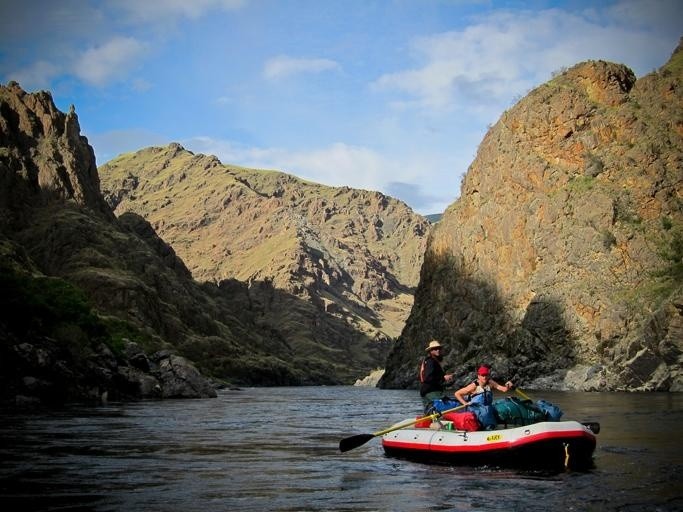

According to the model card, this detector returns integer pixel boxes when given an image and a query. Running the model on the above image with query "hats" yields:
[424,340,445,352]
[477,367,489,376]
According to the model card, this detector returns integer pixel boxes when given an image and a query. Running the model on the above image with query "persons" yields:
[455,366,514,407]
[420,340,453,415]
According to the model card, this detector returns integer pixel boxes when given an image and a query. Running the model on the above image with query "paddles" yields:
[339,405,468,452]
[580,422,600,433]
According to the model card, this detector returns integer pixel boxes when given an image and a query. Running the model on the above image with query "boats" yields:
[382,418,597,469]
